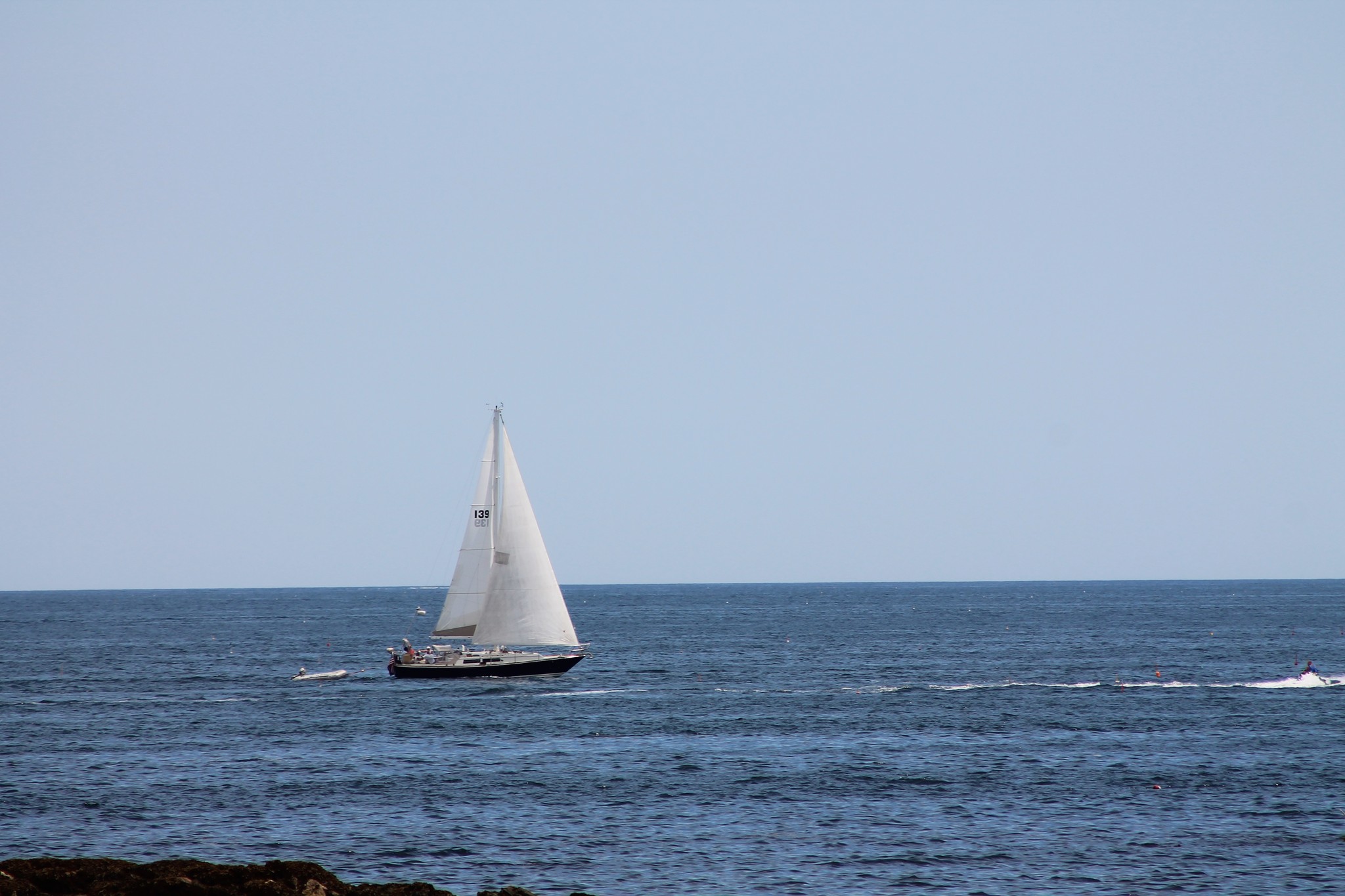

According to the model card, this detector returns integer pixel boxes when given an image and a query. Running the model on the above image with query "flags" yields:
[387,652,395,676]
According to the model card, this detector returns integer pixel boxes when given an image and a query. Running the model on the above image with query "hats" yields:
[427,646,430,649]
[386,648,393,651]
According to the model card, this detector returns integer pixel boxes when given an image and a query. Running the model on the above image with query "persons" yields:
[401,645,438,664]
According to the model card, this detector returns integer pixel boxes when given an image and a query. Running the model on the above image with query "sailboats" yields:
[290,403,586,681]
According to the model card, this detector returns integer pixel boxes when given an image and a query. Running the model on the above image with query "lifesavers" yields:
[402,638,410,650]
[513,650,522,654]
